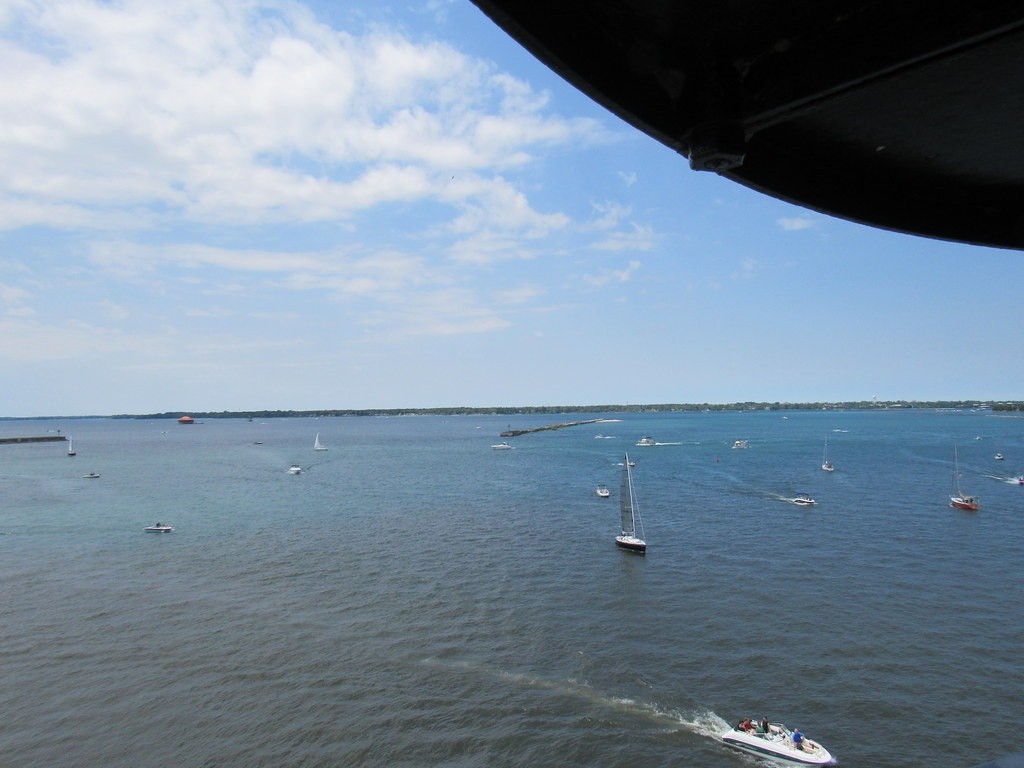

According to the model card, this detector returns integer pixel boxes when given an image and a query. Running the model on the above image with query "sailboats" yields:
[949,442,980,511]
[822,432,834,472]
[313,431,330,452]
[68,427,77,455]
[615,450,648,553]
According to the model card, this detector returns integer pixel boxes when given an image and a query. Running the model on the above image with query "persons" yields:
[791,728,804,750]
[739,720,746,732]
[743,718,751,732]
[760,716,772,733]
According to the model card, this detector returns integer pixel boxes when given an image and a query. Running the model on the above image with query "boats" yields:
[179,414,195,424]
[1019,475,1024,485]
[144,522,173,533]
[82,472,100,478]
[597,483,609,497]
[634,436,656,447]
[790,492,815,505]
[253,442,262,444]
[491,441,511,450]
[730,439,752,450]
[288,464,302,474]
[994,453,1004,460]
[720,716,834,768]
